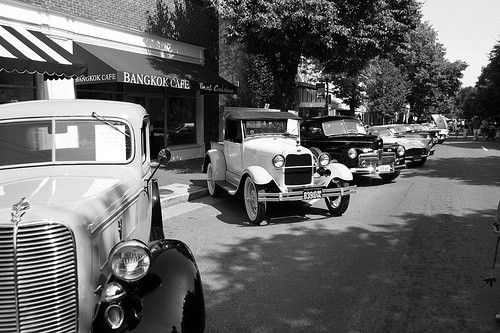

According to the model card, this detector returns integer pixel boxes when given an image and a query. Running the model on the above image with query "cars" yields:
[300,115,405,183]
[367,123,448,168]
[201,107,356,226]
[0,100,207,333]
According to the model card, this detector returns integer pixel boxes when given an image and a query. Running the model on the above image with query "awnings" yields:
[73,40,237,96]
[0,24,88,81]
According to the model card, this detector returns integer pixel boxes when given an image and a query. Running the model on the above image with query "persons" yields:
[492,198,500,322]
[447,116,499,142]
